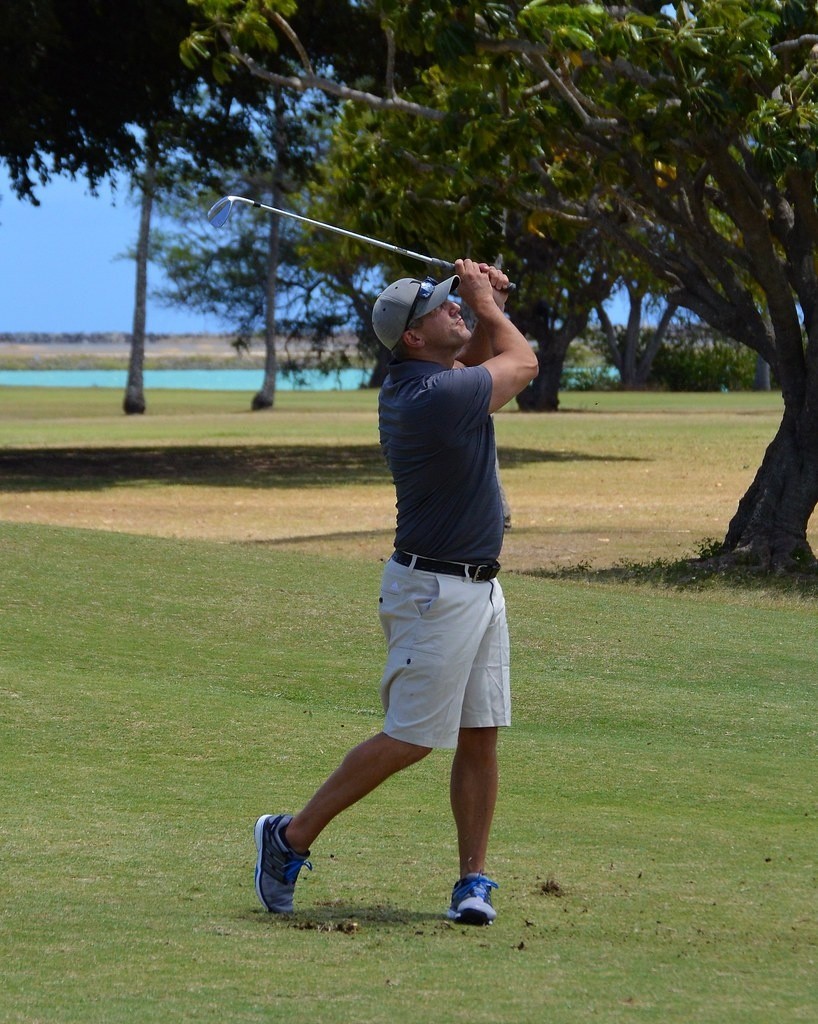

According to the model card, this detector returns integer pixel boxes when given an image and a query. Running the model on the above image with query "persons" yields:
[254,259,539,925]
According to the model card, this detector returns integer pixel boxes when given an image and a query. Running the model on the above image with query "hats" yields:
[372,275,461,351]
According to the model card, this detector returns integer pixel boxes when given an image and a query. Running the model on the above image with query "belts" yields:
[392,550,501,583]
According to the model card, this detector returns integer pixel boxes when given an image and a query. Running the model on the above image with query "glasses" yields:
[405,276,438,331]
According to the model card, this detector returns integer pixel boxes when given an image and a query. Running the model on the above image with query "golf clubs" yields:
[205,193,517,295]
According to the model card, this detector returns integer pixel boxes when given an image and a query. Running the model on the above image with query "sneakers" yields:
[253,813,312,913]
[445,871,498,923]
